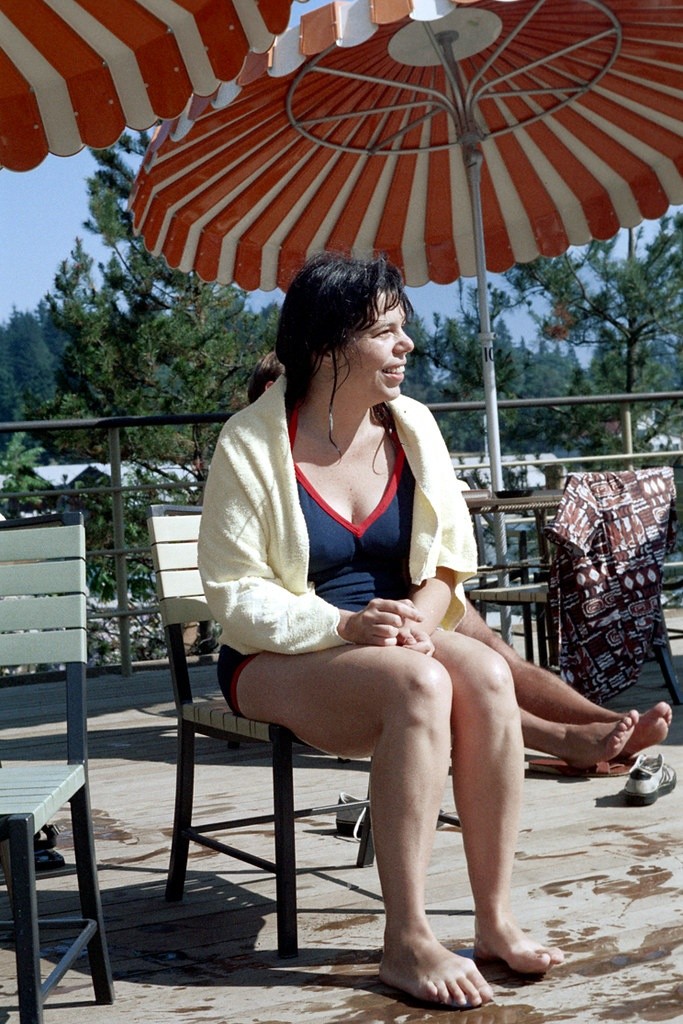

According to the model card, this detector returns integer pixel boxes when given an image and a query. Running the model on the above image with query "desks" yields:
[465,490,566,669]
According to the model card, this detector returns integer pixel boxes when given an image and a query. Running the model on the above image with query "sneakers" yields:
[624,754,677,806]
[335,794,369,837]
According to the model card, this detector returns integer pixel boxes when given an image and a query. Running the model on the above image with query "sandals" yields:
[33,824,66,871]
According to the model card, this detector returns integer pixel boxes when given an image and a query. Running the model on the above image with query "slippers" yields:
[529,756,629,777]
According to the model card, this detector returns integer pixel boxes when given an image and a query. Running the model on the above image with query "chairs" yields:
[146,504,374,959]
[0,511,114,1024]
[470,466,683,706]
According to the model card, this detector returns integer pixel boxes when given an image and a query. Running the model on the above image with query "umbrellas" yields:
[0,0,309,173]
[125,0,683,646]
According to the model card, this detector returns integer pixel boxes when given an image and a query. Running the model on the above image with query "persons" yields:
[450,590,674,768]
[196,249,564,1007]
